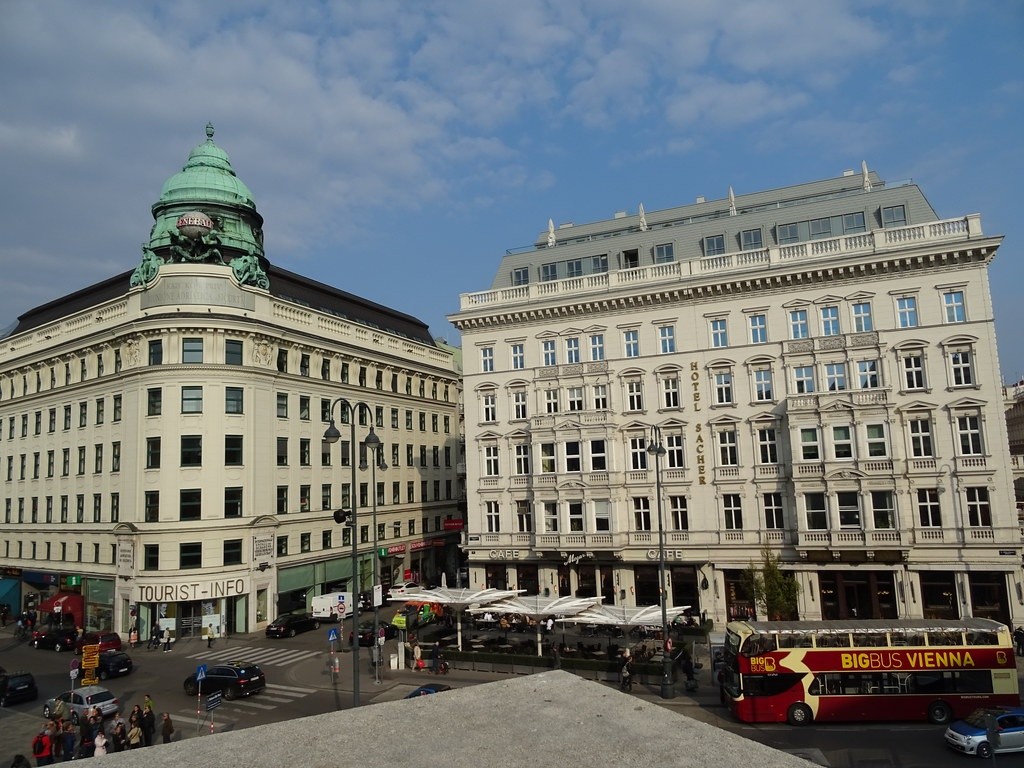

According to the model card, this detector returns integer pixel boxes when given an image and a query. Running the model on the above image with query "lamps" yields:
[701,578,709,589]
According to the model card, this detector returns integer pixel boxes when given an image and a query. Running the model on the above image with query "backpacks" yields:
[33,735,47,753]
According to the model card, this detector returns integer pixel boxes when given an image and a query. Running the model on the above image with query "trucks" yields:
[315,593,363,621]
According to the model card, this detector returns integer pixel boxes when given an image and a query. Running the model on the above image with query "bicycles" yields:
[15,620,30,638]
[147,631,161,650]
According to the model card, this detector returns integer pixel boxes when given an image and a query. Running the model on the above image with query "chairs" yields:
[748,636,993,658]
[489,635,507,653]
[462,634,467,643]
[577,640,602,655]
[472,634,478,638]
[528,638,535,648]
[545,638,550,642]
[499,623,505,632]
[546,640,556,650]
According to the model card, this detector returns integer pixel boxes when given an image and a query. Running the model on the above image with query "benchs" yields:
[607,644,623,659]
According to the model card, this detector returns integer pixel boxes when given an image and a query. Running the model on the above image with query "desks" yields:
[507,637,520,643]
[447,644,459,650]
[586,625,598,638]
[478,634,493,640]
[441,634,457,641]
[649,655,664,663]
[511,621,519,631]
[476,618,499,631]
[592,650,607,658]
[659,648,664,651]
[582,642,598,649]
[616,647,626,657]
[472,644,485,649]
[542,641,551,645]
[655,651,664,655]
[469,639,483,643]
[620,642,636,653]
[498,644,512,649]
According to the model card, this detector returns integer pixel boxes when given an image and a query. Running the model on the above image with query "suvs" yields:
[183,661,266,700]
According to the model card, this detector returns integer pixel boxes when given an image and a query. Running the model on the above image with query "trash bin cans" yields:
[372,644,379,662]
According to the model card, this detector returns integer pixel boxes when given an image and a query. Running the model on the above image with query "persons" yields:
[160,603,168,618]
[0,604,9,627]
[436,603,452,625]
[484,613,554,634]
[163,627,172,653]
[207,623,214,648]
[75,625,84,641]
[411,641,426,672]
[31,695,155,766]
[146,621,162,649]
[238,246,261,286]
[162,713,174,744]
[620,656,634,695]
[551,646,561,670]
[1013,626,1024,657]
[685,613,694,626]
[138,243,156,289]
[46,613,64,632]
[432,641,443,673]
[22,608,37,634]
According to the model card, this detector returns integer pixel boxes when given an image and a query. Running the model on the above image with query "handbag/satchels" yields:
[126,740,130,745]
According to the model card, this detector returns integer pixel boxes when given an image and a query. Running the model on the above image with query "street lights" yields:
[324,399,389,709]
[645,423,677,699]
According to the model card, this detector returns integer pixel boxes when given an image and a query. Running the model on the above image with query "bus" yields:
[719,618,1021,727]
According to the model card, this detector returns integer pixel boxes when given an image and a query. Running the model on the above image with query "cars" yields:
[358,591,390,611]
[265,614,320,638]
[404,683,452,699]
[43,684,119,726]
[944,705,1024,759]
[1,668,39,708]
[391,602,445,630]
[348,619,399,648]
[29,629,132,681]
[389,581,426,598]
